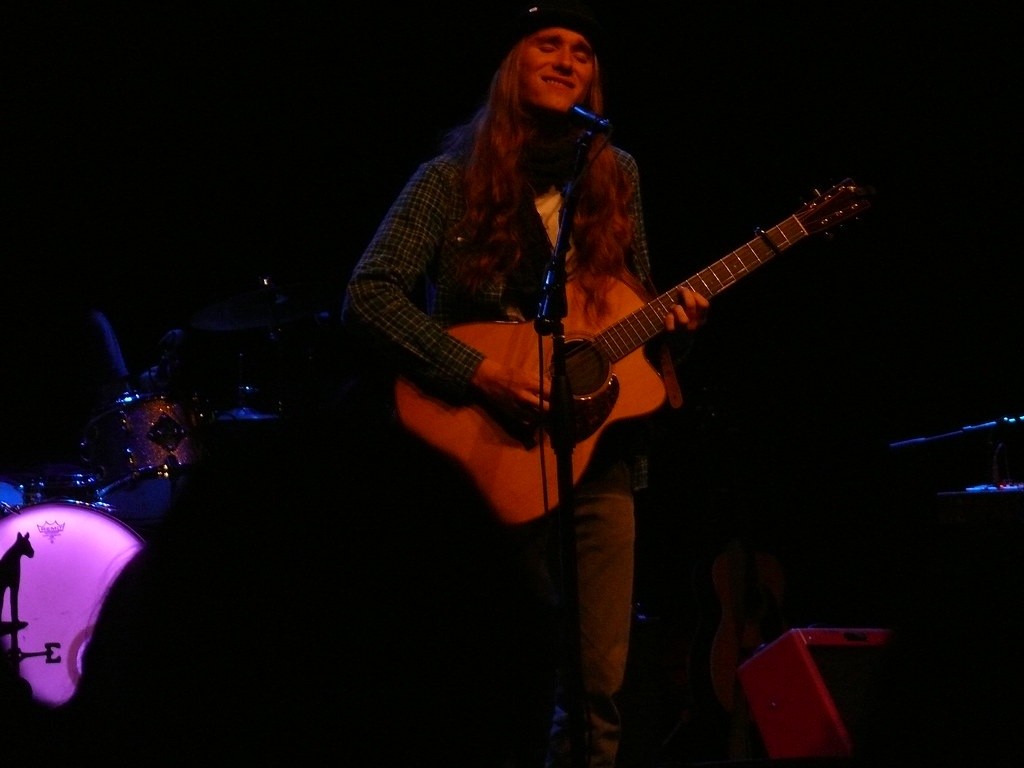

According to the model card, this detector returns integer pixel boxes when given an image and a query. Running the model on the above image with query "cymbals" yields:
[199,407,282,434]
[189,301,311,332]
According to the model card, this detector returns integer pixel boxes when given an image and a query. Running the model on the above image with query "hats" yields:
[495,0,604,65]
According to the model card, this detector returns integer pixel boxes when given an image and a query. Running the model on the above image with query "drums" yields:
[0,465,101,515]
[1,501,150,716]
[80,397,198,530]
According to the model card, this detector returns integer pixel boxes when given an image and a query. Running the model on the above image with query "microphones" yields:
[567,102,611,135]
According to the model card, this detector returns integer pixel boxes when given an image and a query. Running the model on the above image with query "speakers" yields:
[738,628,892,761]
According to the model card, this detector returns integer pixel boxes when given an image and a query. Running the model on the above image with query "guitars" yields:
[691,540,787,716]
[393,175,874,527]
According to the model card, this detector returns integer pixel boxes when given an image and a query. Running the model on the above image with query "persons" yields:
[341,0,710,768]
